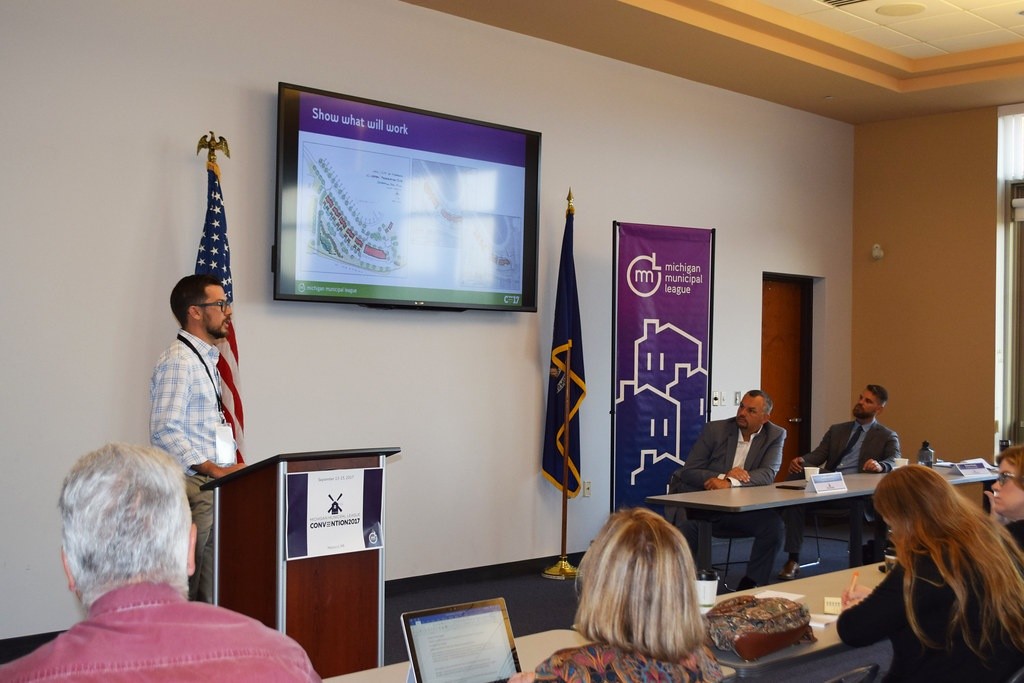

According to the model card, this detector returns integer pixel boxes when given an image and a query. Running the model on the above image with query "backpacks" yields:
[700,595,818,663]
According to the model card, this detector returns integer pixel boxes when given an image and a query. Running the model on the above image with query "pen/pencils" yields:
[848,571,859,594]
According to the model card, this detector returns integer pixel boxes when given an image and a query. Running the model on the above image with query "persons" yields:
[993,445,1024,551]
[148,274,248,605]
[671,390,787,596]
[778,383,901,581]
[0,443,321,682]
[506,506,725,683]
[837,464,1024,683]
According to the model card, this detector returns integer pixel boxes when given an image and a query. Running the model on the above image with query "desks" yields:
[322,463,998,683]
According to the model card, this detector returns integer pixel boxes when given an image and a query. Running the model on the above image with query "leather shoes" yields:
[777,559,800,580]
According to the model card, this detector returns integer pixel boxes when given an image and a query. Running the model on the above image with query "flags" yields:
[542,214,588,498]
[194,156,245,465]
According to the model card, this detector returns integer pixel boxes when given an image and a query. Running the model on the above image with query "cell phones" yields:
[775,485,805,490]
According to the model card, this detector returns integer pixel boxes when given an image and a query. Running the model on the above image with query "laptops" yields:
[401,596,522,683]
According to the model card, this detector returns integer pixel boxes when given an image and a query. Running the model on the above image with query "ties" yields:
[828,425,863,472]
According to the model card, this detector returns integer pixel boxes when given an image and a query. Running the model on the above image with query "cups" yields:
[695,570,719,615]
[999,440,1011,452]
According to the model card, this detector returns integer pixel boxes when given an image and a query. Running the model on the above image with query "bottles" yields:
[916,440,934,469]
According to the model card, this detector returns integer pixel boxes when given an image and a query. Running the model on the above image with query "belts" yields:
[195,472,215,479]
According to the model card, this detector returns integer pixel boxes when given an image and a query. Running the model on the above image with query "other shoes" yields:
[736,576,759,592]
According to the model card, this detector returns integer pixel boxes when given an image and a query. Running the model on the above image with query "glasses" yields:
[996,472,1024,486]
[197,300,230,312]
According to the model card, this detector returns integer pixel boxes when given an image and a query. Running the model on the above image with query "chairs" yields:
[798,505,853,569]
[711,523,756,591]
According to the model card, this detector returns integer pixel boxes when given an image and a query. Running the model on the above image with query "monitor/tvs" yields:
[274,82,541,312]
